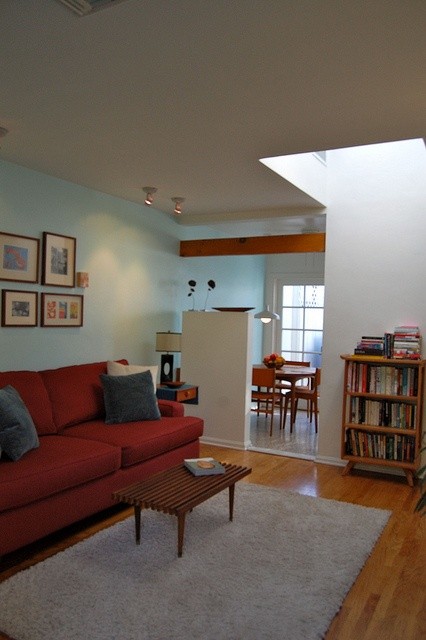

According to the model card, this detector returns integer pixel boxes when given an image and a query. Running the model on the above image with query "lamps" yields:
[255,253,284,325]
[155,330,181,383]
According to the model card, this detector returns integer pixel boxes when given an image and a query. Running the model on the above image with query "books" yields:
[346,396,417,432]
[354,324,423,359]
[183,456,226,477]
[345,429,416,464]
[347,361,418,397]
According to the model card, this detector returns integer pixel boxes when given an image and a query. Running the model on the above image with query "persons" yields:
[185,457,215,469]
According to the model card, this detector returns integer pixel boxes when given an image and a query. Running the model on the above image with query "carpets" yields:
[1,476,394,638]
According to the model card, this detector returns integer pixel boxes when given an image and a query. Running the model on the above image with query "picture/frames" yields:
[41,231,77,288]
[41,292,84,328]
[0,232,41,283]
[1,289,37,327]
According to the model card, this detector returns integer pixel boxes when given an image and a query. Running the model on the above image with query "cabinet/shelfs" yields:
[340,355,426,487]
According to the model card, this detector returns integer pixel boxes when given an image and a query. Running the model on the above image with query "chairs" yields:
[281,365,320,434]
[251,367,281,437]
[266,361,309,425]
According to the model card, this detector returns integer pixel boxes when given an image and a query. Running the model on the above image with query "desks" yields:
[158,385,198,401]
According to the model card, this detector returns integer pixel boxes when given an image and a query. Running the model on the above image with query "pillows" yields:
[106,358,158,394]
[99,370,161,422]
[2,384,40,462]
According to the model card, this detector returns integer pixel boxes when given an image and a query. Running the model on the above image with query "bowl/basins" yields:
[162,380,185,389]
[261,361,286,370]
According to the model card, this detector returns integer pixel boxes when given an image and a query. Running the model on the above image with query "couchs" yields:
[1,359,205,555]
